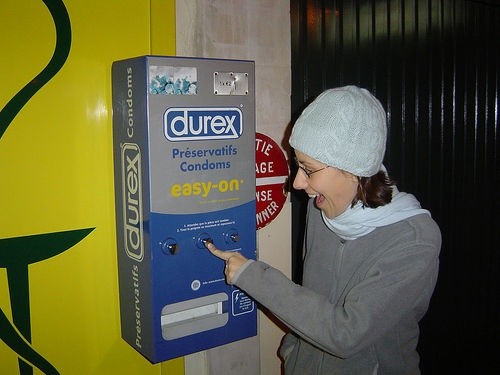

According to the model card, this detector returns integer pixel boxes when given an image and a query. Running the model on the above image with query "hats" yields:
[289,85,387,177]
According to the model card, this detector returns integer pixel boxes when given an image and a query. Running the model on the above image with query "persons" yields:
[208,86,441,375]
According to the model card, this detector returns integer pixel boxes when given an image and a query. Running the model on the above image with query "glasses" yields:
[295,157,330,178]
